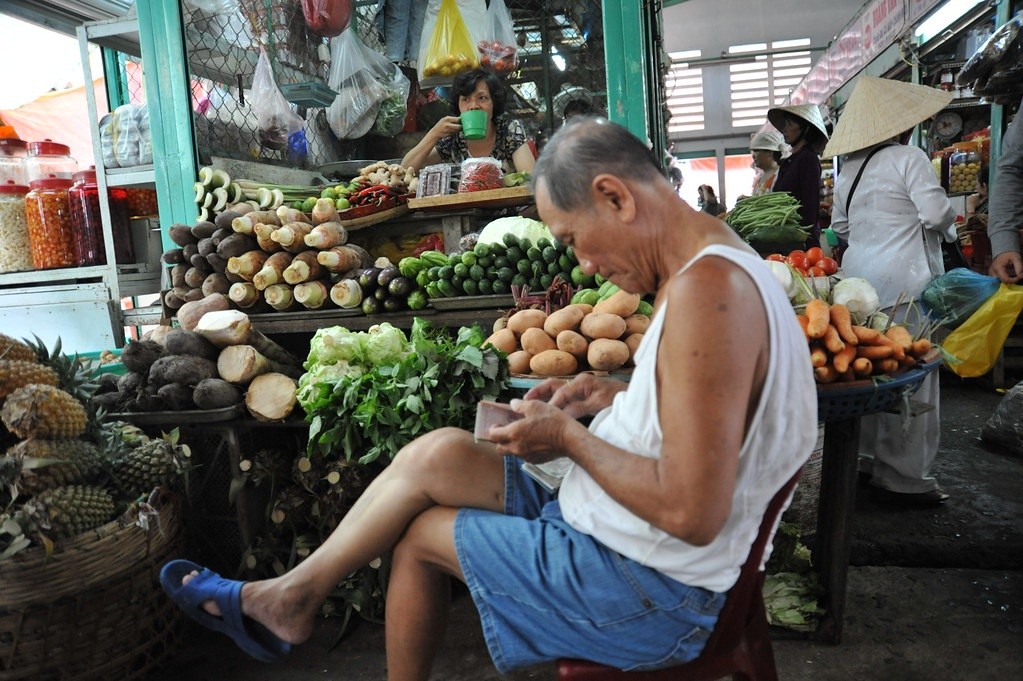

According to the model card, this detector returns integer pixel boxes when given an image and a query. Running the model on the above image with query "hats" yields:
[820,73,954,159]
[767,102,830,154]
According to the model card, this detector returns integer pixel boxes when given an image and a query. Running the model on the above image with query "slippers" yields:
[975,426,1023,458]
[158,558,292,666]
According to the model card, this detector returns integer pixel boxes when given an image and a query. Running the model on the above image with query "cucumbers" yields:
[429,233,582,293]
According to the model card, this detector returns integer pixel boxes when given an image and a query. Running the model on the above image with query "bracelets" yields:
[966,211,975,214]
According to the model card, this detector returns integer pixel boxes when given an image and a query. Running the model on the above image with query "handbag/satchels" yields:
[920,267,1002,331]
[250,42,304,150]
[457,157,505,193]
[324,26,411,141]
[939,282,1023,378]
[416,0,519,89]
[300,0,352,36]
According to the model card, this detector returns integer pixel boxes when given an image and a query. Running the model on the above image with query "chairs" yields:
[555,459,813,681]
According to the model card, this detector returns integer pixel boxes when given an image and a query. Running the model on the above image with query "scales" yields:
[318,159,379,184]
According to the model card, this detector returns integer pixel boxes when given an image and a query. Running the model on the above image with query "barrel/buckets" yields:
[129,215,164,264]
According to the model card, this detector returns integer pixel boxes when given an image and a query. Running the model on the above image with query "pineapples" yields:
[0,333,188,554]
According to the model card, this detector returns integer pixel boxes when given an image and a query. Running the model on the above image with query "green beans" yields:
[725,191,814,244]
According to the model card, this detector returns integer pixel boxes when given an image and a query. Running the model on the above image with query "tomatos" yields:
[477,42,517,72]
[767,247,838,277]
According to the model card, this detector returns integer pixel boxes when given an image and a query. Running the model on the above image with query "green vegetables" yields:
[298,316,512,467]
[372,92,407,137]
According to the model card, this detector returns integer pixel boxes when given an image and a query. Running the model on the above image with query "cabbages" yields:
[832,277,880,322]
[761,261,798,301]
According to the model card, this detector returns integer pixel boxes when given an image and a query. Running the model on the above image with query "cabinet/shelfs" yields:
[75,12,170,327]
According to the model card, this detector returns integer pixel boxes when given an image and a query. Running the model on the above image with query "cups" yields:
[458,109,488,140]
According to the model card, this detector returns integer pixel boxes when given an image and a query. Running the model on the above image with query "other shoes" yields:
[855,473,950,510]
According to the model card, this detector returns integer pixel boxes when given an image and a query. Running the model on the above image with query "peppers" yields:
[342,179,405,207]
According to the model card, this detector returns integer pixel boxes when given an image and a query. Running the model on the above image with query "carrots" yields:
[795,300,930,380]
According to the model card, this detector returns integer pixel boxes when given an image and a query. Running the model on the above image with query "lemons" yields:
[291,183,363,211]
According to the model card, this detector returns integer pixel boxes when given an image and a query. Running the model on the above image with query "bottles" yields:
[25,174,84,271]
[69,165,136,267]
[0,139,78,186]
[930,128,990,193]
[0,179,29,274]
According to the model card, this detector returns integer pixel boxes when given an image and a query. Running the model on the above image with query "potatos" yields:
[481,289,655,375]
[423,55,479,76]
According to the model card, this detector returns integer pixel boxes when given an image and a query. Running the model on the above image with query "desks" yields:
[769,360,947,645]
[407,185,533,254]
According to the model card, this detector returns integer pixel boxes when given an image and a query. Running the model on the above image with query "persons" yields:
[819,75,958,506]
[940,163,993,388]
[737,104,829,253]
[400,68,536,219]
[988,97,1023,284]
[698,184,727,219]
[161,115,818,681]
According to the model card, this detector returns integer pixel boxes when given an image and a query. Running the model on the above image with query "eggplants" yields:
[359,267,414,316]
